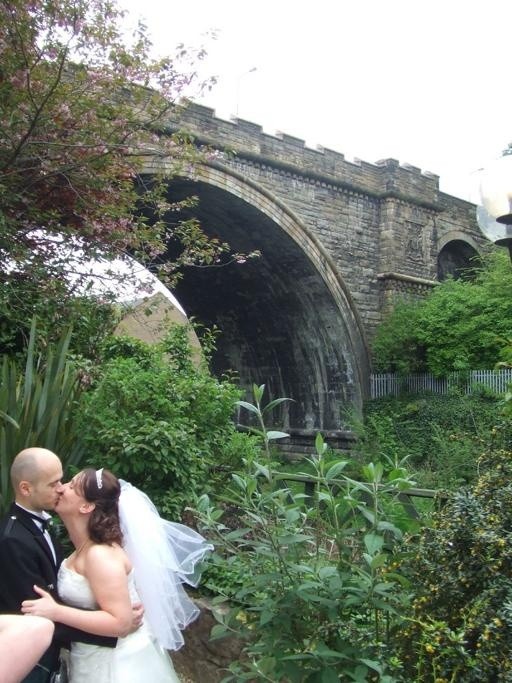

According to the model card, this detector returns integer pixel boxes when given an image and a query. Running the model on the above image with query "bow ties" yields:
[43,517,53,534]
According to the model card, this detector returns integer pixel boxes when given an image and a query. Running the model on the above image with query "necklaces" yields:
[76,537,90,556]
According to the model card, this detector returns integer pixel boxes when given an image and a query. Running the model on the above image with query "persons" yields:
[0,447,145,683]
[21,467,214,683]
[0,614,55,683]
